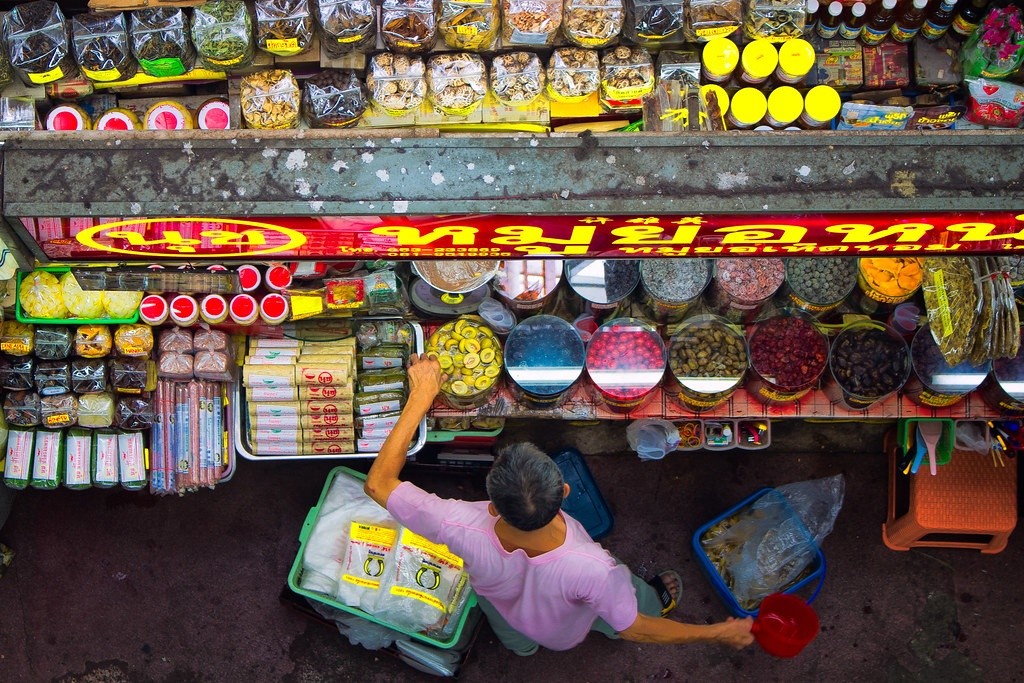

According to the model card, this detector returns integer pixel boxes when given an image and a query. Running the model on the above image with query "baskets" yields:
[16,267,140,324]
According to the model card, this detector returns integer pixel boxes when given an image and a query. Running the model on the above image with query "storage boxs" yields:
[16,265,139,326]
[216,317,505,482]
[551,448,613,542]
[692,486,828,622]
[289,466,480,650]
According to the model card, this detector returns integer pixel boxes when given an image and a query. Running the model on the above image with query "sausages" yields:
[148,378,223,495]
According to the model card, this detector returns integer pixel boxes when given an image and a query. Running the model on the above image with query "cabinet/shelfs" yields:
[0,0,1023,449]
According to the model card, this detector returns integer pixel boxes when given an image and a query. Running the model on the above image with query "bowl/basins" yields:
[750,593,819,659]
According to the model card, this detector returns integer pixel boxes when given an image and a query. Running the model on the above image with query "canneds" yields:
[700,36,841,128]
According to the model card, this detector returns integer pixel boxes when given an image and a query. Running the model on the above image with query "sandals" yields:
[605,570,683,640]
[512,642,539,657]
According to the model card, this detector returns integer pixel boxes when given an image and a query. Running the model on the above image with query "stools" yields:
[880,426,1018,555]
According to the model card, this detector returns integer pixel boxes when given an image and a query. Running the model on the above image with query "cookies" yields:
[367,46,655,112]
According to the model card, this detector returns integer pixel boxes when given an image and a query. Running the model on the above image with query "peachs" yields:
[428,319,502,396]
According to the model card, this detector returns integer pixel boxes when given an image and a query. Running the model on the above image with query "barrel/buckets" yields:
[413,255,1024,415]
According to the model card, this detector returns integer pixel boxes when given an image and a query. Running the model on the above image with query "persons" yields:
[363,354,755,656]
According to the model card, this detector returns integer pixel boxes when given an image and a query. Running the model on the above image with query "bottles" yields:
[804,0,988,47]
[139,265,292,327]
[43,97,231,131]
